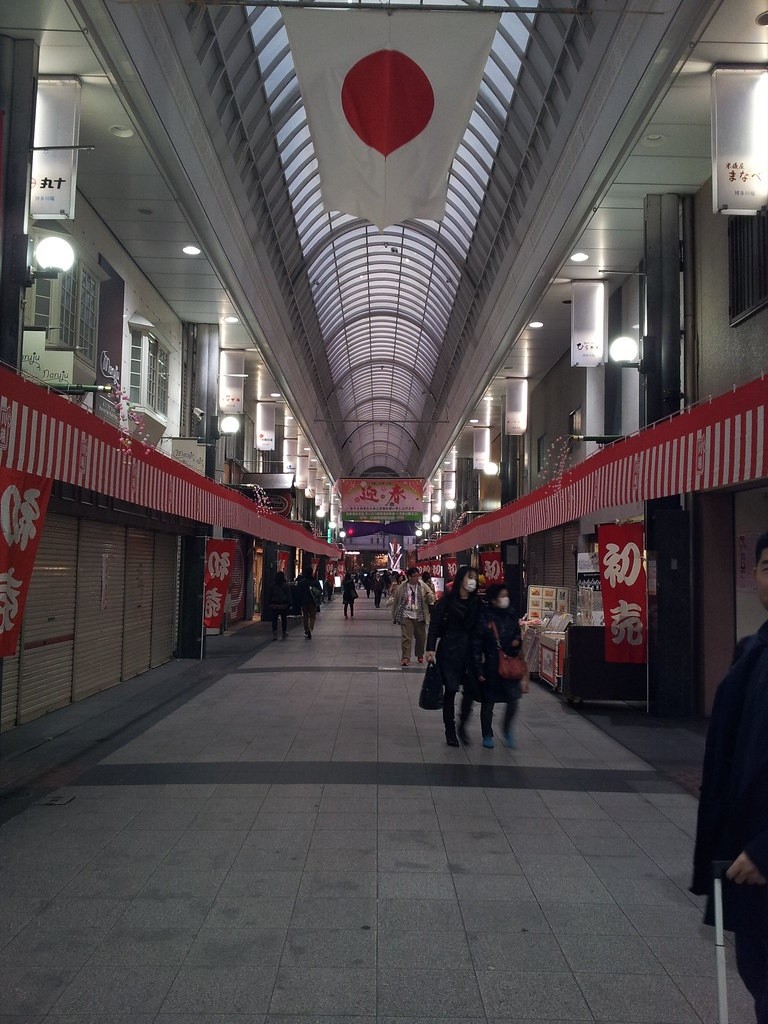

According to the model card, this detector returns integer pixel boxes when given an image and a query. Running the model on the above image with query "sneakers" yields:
[417,658,428,666]
[400,658,410,669]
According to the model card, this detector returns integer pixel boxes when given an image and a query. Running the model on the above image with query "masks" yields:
[497,597,510,609]
[465,579,476,593]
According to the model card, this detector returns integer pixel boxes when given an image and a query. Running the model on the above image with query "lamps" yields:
[25,235,76,289]
[609,335,657,374]
[210,415,240,441]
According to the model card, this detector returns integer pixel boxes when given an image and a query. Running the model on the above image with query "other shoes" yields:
[305,631,311,640]
[282,632,288,639]
[483,731,494,749]
[273,636,277,640]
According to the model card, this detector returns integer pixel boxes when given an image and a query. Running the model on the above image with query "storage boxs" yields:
[576,552,607,626]
[420,576,445,605]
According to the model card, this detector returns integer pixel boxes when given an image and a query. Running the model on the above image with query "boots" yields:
[445,721,459,747]
[458,721,471,745]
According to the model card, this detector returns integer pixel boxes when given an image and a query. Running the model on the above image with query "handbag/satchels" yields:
[498,651,525,680]
[520,663,529,693]
[419,661,444,709]
[351,589,358,599]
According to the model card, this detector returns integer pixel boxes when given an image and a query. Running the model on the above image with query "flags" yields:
[276,551,290,573]
[598,523,647,664]
[325,560,346,575]
[310,559,321,574]
[478,551,502,584]
[204,539,237,636]
[0,463,56,658]
[415,557,458,580]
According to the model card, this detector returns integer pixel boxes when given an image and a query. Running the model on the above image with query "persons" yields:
[297,567,323,639]
[265,571,294,641]
[424,566,486,749]
[688,532,768,1024]
[468,582,523,751]
[326,568,436,667]
